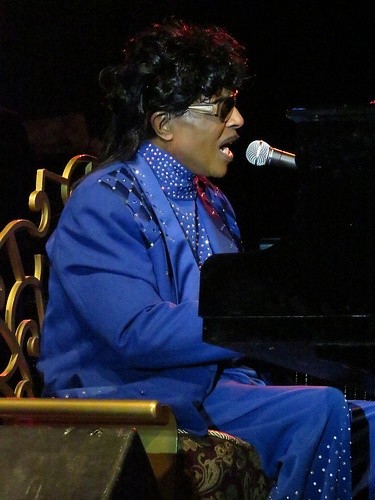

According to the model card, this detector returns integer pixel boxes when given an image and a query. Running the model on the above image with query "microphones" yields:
[246,140,295,167]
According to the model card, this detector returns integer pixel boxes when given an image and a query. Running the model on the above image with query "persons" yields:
[38,16,375,500]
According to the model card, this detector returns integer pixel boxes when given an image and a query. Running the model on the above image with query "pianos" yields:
[199,251,375,347]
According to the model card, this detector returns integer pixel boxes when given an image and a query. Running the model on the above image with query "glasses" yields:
[190,94,239,124]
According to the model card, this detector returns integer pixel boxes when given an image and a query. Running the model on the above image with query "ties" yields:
[193,175,241,254]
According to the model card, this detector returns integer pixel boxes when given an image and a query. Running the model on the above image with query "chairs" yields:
[1,153,260,500]
